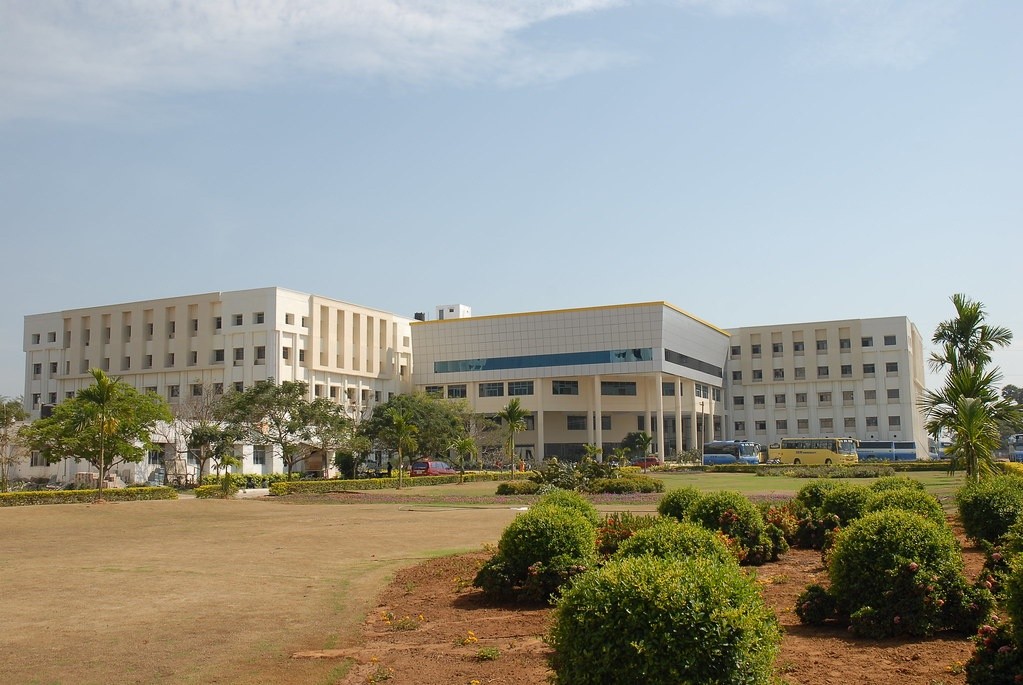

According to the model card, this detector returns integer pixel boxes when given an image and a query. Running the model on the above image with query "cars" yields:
[409,459,457,477]
[631,457,661,467]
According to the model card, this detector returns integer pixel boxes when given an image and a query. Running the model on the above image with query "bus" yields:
[759,445,767,463]
[1008,434,1023,462]
[768,443,780,460]
[779,437,860,466]
[939,442,955,458]
[929,446,938,460]
[703,439,762,467]
[857,441,918,464]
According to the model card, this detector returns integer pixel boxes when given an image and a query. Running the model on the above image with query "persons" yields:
[387,462,393,478]
[375,463,383,477]
